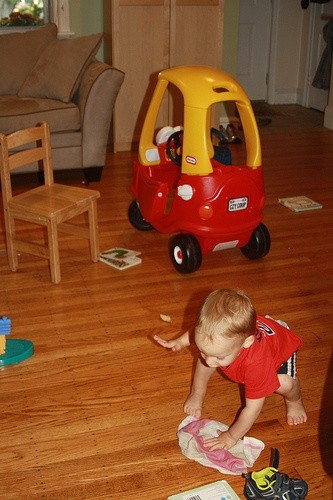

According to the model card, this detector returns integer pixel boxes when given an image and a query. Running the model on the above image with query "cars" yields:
[128,66,272,276]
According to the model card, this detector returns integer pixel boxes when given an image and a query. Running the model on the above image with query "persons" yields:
[153,288,308,452]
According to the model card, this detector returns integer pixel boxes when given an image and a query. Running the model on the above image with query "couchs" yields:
[0,23,124,183]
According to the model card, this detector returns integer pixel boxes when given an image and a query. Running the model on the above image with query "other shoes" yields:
[220,121,242,143]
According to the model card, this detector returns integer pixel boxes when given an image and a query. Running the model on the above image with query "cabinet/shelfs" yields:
[103,0,225,155]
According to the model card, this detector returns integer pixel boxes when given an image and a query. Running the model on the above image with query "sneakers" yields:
[241,447,307,500]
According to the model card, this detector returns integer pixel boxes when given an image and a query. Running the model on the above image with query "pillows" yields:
[20,32,106,103]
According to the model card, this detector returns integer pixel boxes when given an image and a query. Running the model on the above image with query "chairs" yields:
[0,122,102,282]
